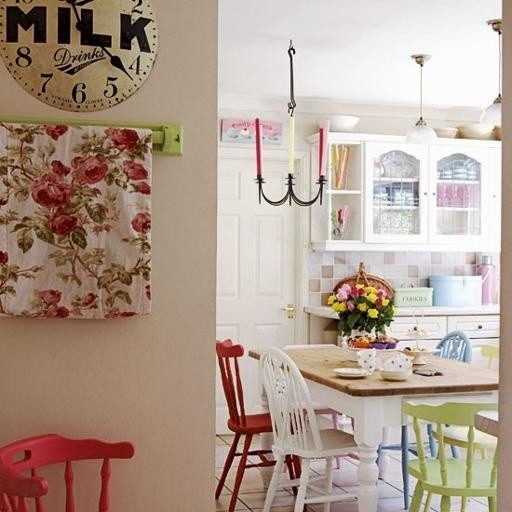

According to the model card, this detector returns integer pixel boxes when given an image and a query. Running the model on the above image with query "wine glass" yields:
[436,183,467,209]
[399,348,441,366]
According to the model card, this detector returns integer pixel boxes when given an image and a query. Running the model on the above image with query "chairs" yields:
[376,329,475,509]
[253,343,360,511]
[430,344,500,462]
[2,422,141,512]
[394,394,504,512]
[214,333,317,511]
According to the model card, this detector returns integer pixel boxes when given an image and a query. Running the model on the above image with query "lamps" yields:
[249,37,332,208]
[404,54,442,151]
[480,17,505,130]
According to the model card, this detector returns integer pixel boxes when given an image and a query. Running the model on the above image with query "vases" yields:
[344,328,377,362]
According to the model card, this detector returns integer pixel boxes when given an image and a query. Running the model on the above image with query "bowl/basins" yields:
[379,368,408,381]
[457,122,495,140]
[319,116,360,131]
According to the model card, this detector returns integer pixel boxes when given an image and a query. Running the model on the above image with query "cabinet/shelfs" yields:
[305,130,502,255]
[309,313,450,448]
[445,316,501,373]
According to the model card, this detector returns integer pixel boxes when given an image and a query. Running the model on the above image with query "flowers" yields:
[322,285,399,345]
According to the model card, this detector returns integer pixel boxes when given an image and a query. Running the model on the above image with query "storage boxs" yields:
[394,288,436,308]
[424,274,484,308]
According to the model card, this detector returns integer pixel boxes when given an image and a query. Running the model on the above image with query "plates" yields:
[331,367,372,379]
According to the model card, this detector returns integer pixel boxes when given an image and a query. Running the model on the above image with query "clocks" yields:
[0,0,162,116]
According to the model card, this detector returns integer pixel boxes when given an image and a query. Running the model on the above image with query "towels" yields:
[0,118,158,325]
